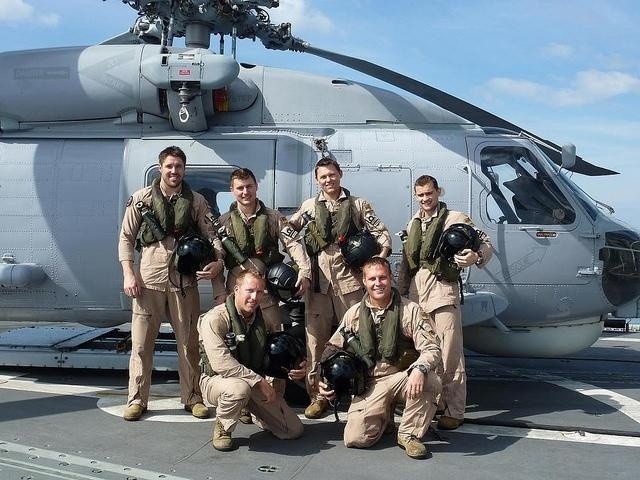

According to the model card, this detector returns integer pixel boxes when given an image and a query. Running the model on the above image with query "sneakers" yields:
[185,401,211,418]
[397,433,428,458]
[214,418,234,451]
[124,404,145,421]
[438,416,465,430]
[240,407,253,424]
[305,392,329,418]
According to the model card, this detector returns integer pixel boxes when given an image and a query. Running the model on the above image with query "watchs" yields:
[414,364,427,374]
[474,251,482,265]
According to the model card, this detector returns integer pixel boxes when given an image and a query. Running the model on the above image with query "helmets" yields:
[260,331,309,381]
[440,223,482,263]
[267,262,299,290]
[322,348,371,396]
[173,232,217,278]
[341,231,379,269]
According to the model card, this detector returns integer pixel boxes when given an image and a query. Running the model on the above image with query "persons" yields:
[395,175,493,431]
[289,157,392,419]
[197,269,308,451]
[118,145,227,422]
[211,169,312,399]
[318,257,442,458]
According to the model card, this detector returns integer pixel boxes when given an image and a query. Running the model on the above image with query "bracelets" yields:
[218,257,225,269]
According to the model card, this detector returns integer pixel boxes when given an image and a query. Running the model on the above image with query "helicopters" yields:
[0,1,637,373]
